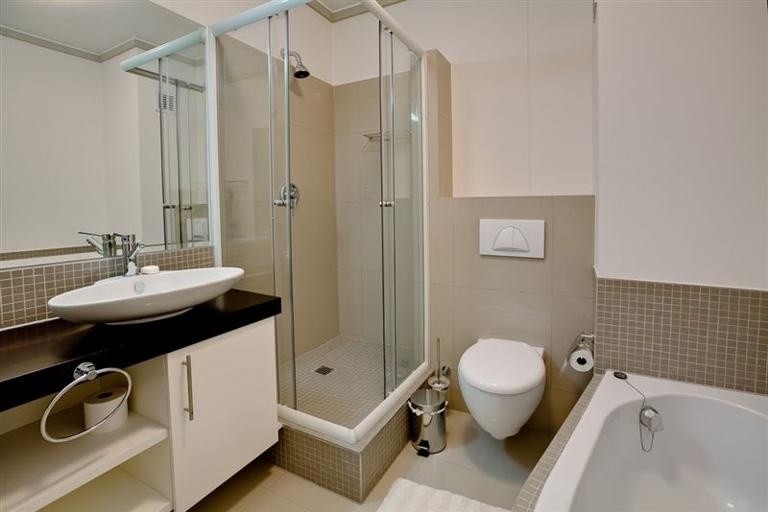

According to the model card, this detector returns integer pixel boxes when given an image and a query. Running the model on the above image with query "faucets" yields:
[79,231,118,256]
[113,233,145,277]
[639,405,665,432]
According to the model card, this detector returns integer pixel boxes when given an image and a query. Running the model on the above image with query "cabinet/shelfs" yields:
[0,297,282,511]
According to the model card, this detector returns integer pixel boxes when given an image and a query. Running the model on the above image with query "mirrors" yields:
[0,1,210,271]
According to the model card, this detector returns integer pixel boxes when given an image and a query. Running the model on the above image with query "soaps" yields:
[140,265,160,274]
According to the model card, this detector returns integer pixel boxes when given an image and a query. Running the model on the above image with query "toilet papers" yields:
[84,386,129,433]
[569,344,594,373]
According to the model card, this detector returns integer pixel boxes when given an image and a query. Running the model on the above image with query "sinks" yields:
[47,267,245,324]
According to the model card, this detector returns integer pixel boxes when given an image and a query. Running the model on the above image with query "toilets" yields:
[457,337,547,440]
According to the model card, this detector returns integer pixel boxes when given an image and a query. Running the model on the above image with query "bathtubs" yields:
[533,369,768,512]
